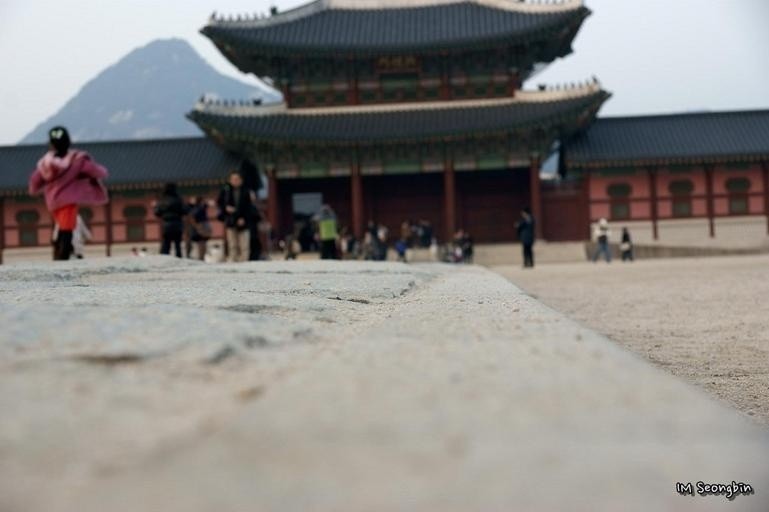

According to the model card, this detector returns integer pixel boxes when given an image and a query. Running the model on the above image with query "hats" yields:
[50,126,70,144]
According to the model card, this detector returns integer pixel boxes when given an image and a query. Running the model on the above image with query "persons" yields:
[591,218,612,263]
[28,126,107,260]
[513,208,537,268]
[153,171,473,263]
[622,228,634,262]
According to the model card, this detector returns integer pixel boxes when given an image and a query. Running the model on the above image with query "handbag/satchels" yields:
[195,221,212,238]
[619,242,632,252]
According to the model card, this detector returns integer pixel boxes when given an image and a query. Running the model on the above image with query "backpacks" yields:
[217,211,222,222]
